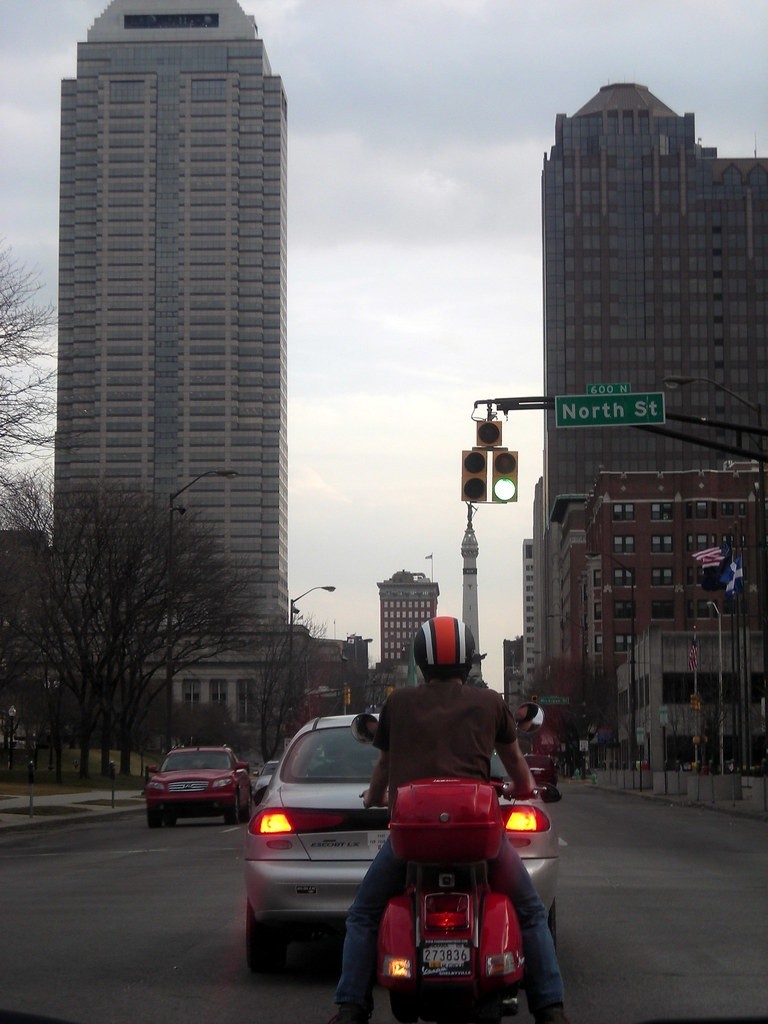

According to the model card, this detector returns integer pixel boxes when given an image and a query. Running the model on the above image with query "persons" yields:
[516,704,539,732]
[356,715,378,742]
[333,617,564,1024]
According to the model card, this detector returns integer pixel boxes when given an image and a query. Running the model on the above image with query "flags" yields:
[689,639,697,670]
[692,544,730,592]
[722,553,743,596]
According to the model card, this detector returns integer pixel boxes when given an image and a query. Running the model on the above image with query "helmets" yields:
[414,617,476,669]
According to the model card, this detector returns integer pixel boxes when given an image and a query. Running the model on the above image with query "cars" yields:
[522,753,562,786]
[239,713,564,980]
[251,761,281,803]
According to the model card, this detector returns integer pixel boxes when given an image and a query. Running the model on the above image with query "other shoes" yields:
[530,1004,572,1024]
[328,1005,370,1024]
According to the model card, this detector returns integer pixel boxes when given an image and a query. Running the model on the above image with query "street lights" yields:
[287,585,338,740]
[705,601,728,775]
[661,374,768,769]
[165,468,241,753]
[547,613,585,707]
[584,553,637,770]
[9,705,17,770]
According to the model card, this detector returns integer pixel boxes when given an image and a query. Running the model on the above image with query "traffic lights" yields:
[690,694,696,710]
[461,450,488,502]
[475,421,502,448]
[492,451,518,502]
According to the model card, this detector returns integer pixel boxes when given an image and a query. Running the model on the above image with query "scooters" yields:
[350,700,547,1024]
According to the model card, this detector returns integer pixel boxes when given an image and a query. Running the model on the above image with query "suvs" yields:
[144,744,251,827]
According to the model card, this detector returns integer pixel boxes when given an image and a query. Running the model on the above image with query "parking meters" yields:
[26,761,38,820]
[109,760,116,808]
